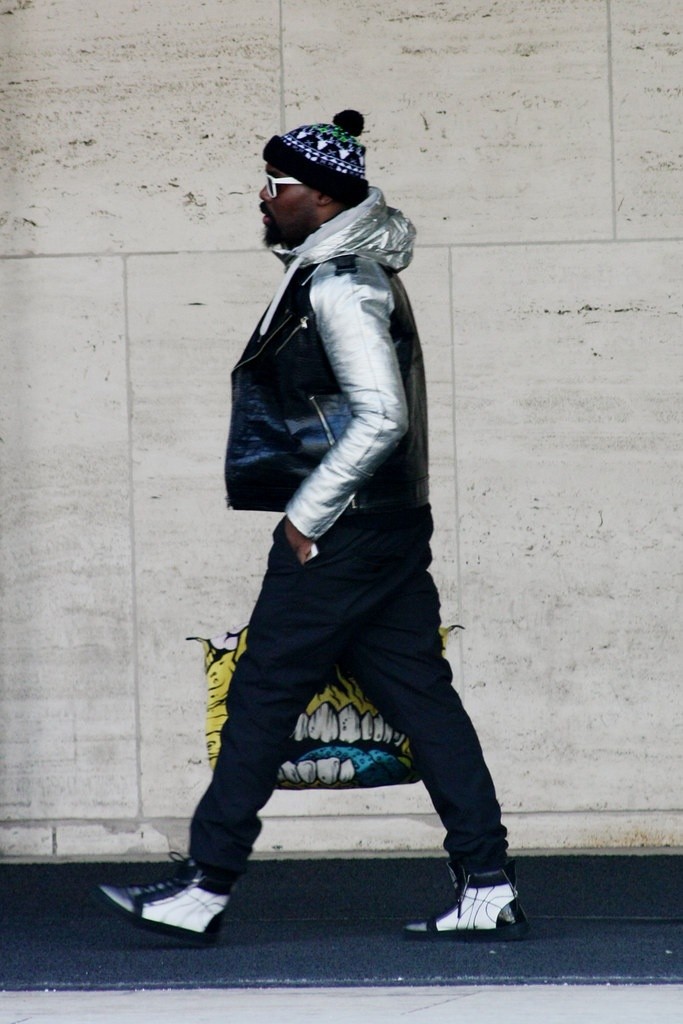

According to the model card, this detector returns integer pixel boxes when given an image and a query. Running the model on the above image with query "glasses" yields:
[266,173,301,198]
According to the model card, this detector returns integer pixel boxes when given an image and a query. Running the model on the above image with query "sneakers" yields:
[97,851,235,945]
[400,862,529,942]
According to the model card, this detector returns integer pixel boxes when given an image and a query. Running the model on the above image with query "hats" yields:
[263,107,369,208]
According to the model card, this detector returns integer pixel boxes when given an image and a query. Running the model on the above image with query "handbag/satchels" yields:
[185,624,476,783]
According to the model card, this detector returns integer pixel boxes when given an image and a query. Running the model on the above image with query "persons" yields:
[88,108,531,949]
